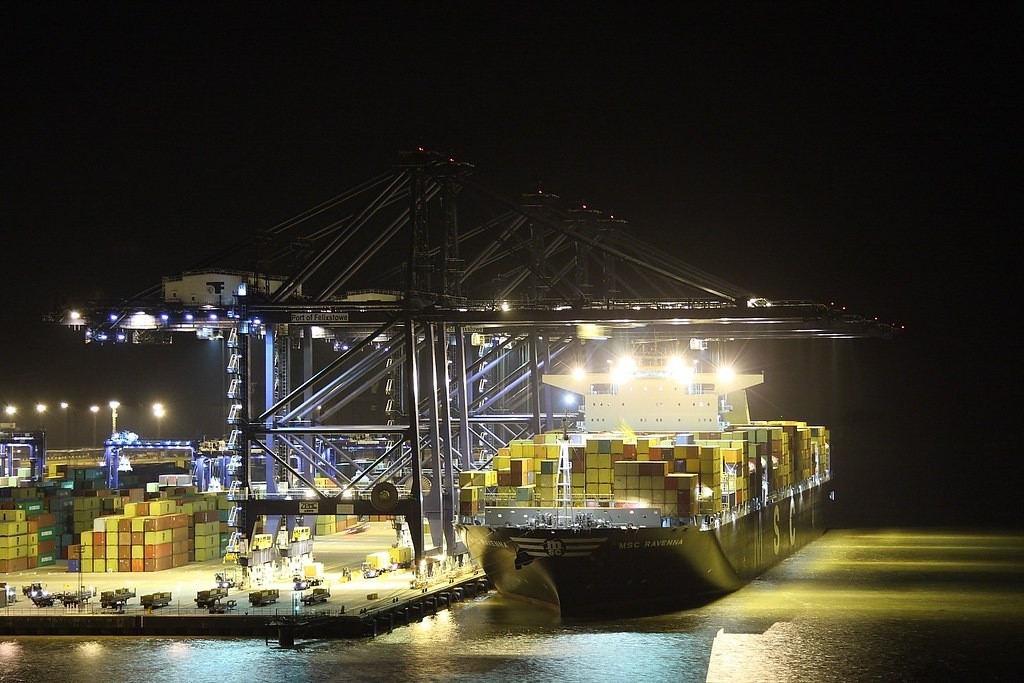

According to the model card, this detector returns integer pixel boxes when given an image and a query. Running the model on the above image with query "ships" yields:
[451,328,835,624]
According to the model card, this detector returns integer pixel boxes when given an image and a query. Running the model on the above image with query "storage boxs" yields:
[250,589,277,597]
[367,594,378,599]
[0,463,266,572]
[143,593,170,601]
[314,588,327,594]
[102,589,125,599]
[367,546,412,570]
[458,421,831,519]
[315,477,339,488]
[305,563,325,580]
[198,588,227,599]
[314,514,431,558]
